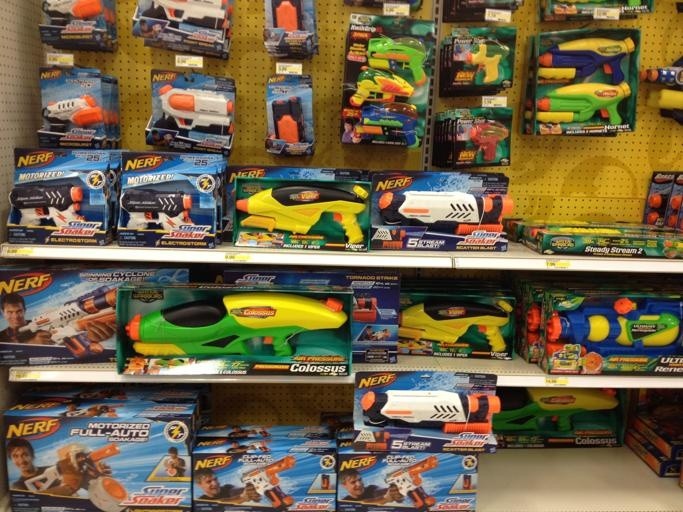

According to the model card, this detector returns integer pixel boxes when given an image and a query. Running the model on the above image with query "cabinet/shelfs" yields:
[0,244,682,510]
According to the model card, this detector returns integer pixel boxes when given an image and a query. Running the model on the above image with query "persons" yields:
[2,289,405,512]
